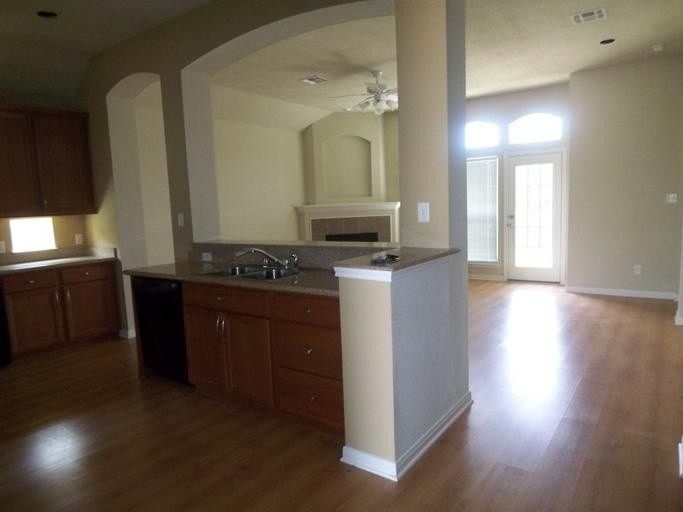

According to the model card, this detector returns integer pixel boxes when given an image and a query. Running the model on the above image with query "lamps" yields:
[598,15,619,48]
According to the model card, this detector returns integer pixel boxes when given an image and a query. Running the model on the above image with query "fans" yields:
[327,70,399,117]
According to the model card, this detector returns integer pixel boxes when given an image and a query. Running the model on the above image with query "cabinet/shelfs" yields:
[182,282,274,409]
[0,102,112,218]
[1,261,125,357]
[270,290,345,435]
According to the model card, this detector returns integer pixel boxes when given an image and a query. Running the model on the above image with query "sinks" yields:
[237,266,311,281]
[195,265,267,276]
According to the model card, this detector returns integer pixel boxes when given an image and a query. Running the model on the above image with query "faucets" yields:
[249,244,287,270]
